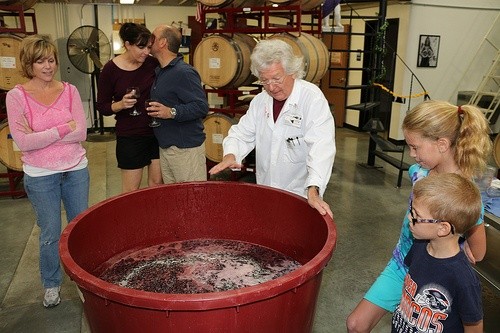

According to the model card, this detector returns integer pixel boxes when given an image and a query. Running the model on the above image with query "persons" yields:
[6,34,92,308]
[147,26,208,183]
[97,21,163,192]
[391,172,484,333]
[208,37,336,221]
[484,135,500,219]
[346,100,493,333]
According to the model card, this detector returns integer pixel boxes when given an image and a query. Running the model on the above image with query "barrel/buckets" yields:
[193,33,261,89]
[201,103,251,164]
[0,113,26,172]
[267,32,330,84]
[0,32,34,91]
[58,180,337,333]
[196,0,328,11]
[0,0,39,11]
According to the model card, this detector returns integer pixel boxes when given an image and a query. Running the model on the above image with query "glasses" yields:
[259,75,286,85]
[410,200,454,234]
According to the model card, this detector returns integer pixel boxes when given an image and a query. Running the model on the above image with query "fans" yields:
[67,26,111,142]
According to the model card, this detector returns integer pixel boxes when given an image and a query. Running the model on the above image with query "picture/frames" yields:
[418,35,440,67]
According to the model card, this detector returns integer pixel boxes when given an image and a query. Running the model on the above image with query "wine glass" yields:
[145,98,162,128]
[126,86,142,116]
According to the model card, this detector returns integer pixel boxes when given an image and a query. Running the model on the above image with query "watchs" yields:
[170,106,176,120]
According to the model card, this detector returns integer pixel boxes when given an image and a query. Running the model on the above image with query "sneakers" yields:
[43,286,61,307]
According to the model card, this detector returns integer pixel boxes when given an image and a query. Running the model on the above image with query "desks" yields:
[470,177,500,297]
